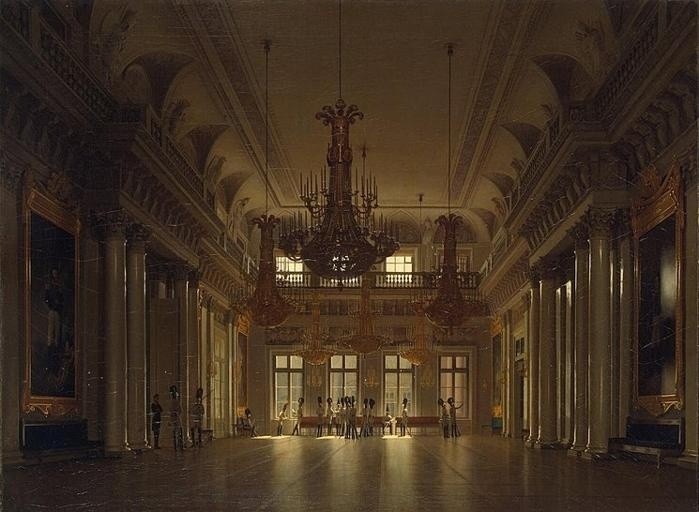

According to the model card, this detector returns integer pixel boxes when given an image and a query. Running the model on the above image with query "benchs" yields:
[607,415,687,470]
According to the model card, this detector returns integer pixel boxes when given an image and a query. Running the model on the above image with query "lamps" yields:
[289,277,338,368]
[396,274,437,368]
[337,269,391,360]
[278,24,402,297]
[406,40,488,338]
[225,37,297,328]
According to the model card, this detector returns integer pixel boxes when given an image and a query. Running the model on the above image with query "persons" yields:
[151,394,164,449]
[188,395,205,449]
[276,395,464,439]
[42,266,65,361]
[166,390,186,451]
[247,411,260,437]
